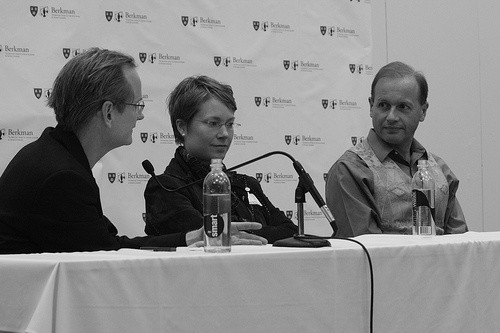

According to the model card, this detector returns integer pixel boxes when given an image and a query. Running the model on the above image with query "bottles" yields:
[202,159,232,253]
[411,160,436,240]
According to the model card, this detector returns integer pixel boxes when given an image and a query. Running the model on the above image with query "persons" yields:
[144,75,299,243]
[325,62,469,238]
[0,48,268,256]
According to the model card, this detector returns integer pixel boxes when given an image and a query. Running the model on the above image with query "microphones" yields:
[141,152,338,231]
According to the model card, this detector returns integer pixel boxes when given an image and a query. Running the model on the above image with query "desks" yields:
[0,229,500,333]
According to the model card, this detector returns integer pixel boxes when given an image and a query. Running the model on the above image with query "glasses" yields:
[188,119,241,130]
[114,101,145,115]
